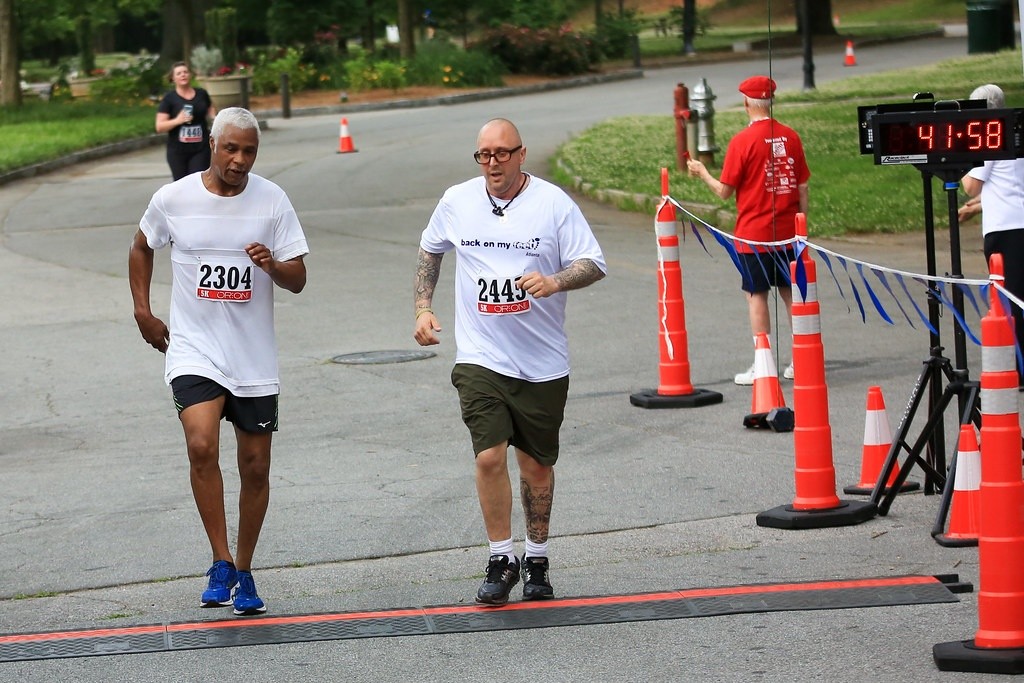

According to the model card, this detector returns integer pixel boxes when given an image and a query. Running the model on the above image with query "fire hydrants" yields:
[687,78,725,167]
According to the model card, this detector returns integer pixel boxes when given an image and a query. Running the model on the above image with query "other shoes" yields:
[783,362,794,378]
[734,364,755,384]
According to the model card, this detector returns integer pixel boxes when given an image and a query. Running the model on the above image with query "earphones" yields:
[492,205,497,214]
[497,210,504,216]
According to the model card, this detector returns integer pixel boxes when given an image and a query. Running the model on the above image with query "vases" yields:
[194,75,251,115]
[67,78,103,100]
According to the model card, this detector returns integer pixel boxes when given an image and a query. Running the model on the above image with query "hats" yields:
[738,76,776,99]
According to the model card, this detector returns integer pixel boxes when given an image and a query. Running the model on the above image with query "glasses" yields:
[474,145,522,164]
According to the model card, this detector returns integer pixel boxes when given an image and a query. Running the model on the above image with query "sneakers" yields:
[200,560,241,608]
[520,553,554,600]
[475,555,520,606]
[232,570,266,615]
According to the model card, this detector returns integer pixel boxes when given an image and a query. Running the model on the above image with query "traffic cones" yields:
[842,41,858,68]
[335,116,359,154]
[840,385,928,496]
[932,422,988,549]
[741,330,798,432]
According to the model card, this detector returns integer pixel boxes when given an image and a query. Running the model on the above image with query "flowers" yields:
[87,67,105,77]
[217,62,248,76]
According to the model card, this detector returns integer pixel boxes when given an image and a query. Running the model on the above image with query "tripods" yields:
[870,158,1024,538]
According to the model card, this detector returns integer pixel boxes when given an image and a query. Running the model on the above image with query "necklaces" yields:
[486,173,526,216]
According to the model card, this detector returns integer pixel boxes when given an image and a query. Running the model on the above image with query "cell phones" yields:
[184,105,193,124]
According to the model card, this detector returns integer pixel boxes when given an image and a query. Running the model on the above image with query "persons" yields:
[960,84,1024,391]
[130,108,309,614]
[688,77,811,384]
[156,62,218,181]
[414,118,606,606]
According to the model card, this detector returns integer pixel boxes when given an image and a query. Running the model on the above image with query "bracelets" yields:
[415,309,432,319]
